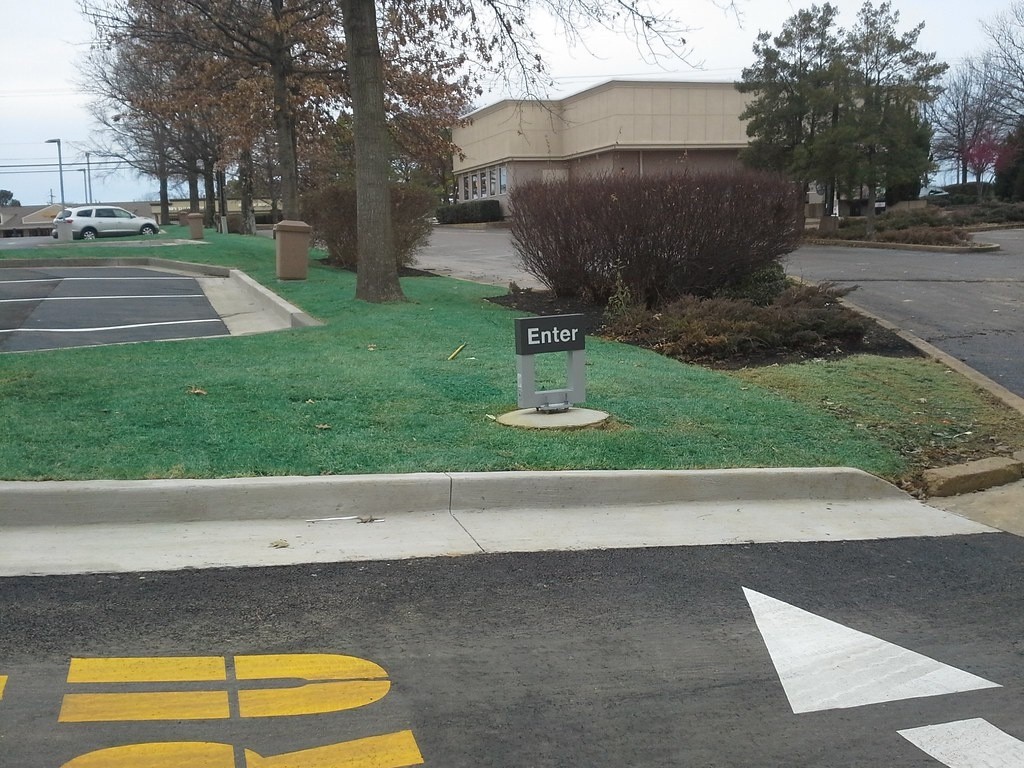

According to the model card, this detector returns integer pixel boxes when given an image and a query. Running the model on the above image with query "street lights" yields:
[77,168,88,205]
[44,138,65,220]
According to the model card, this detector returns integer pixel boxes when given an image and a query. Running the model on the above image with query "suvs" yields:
[52,205,160,240]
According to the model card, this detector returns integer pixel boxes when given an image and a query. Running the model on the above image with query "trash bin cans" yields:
[179,212,188,226]
[57,220,74,240]
[188,212,204,239]
[273,220,312,280]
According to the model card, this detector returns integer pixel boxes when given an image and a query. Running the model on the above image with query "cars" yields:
[919,187,949,201]
